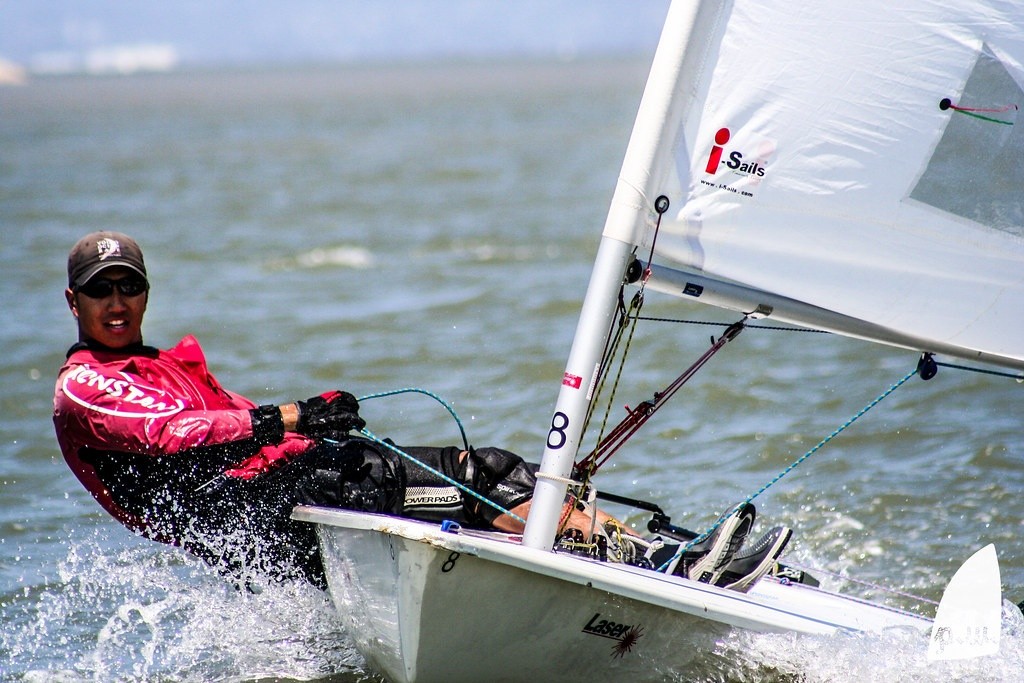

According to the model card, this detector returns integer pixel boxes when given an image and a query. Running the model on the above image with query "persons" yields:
[52,231,793,596]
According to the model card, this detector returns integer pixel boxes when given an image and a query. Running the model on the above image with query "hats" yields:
[65,230,149,288]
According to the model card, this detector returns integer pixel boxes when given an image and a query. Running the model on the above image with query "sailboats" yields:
[289,0,1024,683]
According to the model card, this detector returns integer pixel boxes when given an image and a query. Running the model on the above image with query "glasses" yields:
[72,273,147,298]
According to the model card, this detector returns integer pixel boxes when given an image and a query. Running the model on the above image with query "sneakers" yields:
[715,526,794,593]
[641,500,756,585]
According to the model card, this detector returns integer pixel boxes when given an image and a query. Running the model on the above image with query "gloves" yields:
[293,390,366,434]
